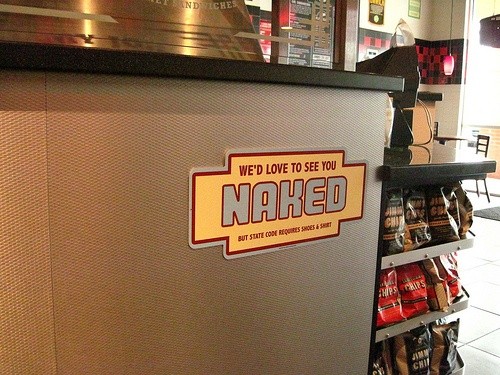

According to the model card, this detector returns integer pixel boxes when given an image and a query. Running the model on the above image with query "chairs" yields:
[472,134,492,204]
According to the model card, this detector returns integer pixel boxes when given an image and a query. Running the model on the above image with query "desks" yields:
[433,135,467,146]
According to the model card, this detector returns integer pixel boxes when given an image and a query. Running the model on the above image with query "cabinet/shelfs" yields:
[366,160,497,375]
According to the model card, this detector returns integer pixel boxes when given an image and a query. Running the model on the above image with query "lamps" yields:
[442,0,455,76]
